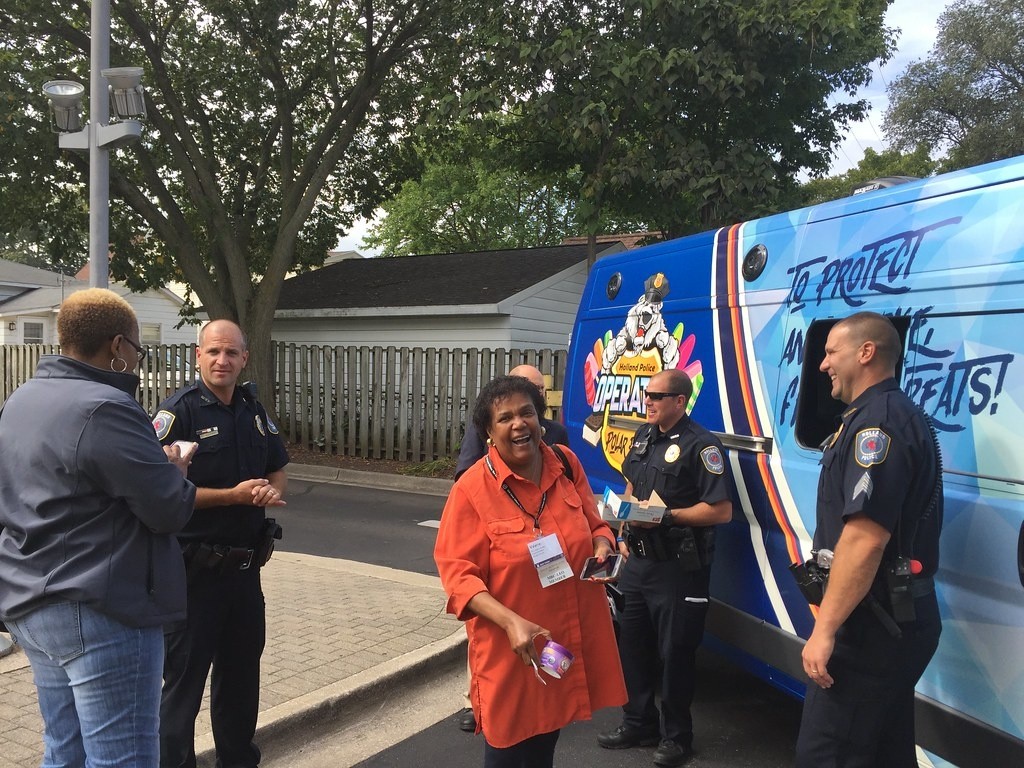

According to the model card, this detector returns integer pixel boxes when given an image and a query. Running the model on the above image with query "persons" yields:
[788,309,944,768]
[152,316,290,768]
[1,288,197,767]
[457,365,574,732]
[435,377,629,766]
[597,369,736,768]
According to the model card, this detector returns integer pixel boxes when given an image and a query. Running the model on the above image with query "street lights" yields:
[42,65,148,293]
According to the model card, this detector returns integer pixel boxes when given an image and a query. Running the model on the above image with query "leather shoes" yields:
[652,739,691,766]
[597,724,662,749]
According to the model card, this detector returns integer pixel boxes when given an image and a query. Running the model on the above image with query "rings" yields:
[811,669,817,673]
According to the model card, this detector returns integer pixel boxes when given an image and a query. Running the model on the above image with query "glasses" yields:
[644,389,680,400]
[115,333,146,363]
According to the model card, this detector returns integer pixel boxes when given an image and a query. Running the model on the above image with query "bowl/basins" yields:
[541,639,577,679]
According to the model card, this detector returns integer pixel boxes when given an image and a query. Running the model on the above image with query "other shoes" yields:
[459,708,476,730]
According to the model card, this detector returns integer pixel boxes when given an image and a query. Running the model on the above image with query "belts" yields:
[651,532,716,552]
[910,576,936,599]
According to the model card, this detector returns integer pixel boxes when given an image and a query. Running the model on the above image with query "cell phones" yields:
[580,554,622,580]
[170,441,193,458]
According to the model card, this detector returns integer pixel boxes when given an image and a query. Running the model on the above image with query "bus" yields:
[561,147,1024,768]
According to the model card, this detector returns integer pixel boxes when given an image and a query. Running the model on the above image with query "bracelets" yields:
[617,537,623,542]
